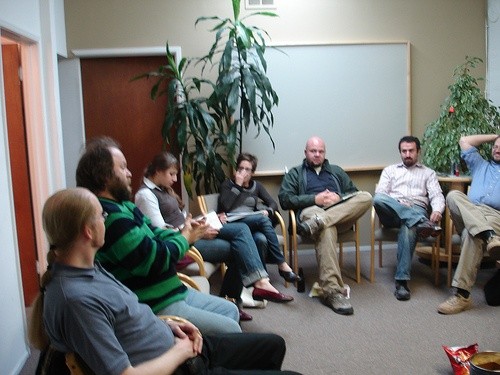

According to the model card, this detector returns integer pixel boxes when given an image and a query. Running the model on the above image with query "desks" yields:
[416,174,495,264]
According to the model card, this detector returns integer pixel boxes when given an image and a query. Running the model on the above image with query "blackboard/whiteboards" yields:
[232,40,412,177]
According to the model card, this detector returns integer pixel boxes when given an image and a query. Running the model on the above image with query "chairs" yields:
[370,206,461,288]
[64,193,360,375]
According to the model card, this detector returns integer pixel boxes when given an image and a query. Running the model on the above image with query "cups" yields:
[469,352,500,375]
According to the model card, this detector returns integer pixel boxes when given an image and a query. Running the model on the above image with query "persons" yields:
[76,135,244,334]
[218,152,301,283]
[134,151,294,320]
[373,136,445,299]
[278,136,373,316]
[438,134,500,315]
[28,187,303,375]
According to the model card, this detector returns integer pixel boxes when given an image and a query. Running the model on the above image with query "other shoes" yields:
[327,291,353,314]
[417,221,442,238]
[394,281,410,300]
[239,310,252,320]
[252,287,294,302]
[278,268,301,283]
[301,216,322,238]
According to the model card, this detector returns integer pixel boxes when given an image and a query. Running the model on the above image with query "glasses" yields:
[239,166,252,171]
[96,211,108,219]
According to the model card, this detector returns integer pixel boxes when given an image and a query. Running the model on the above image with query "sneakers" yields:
[487,235,500,257]
[437,293,472,314]
